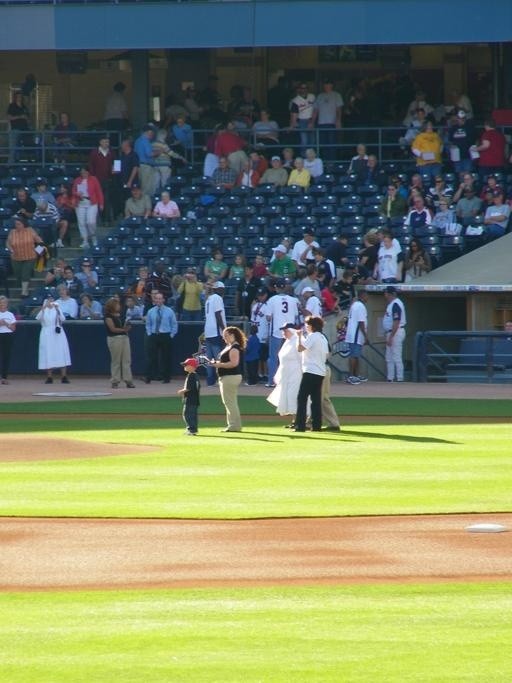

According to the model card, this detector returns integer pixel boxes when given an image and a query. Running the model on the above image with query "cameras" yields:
[185,274,190,279]
[50,302,55,307]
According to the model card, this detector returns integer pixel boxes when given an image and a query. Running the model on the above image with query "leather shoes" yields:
[285,423,339,432]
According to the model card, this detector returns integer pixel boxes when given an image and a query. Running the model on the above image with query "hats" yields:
[272,155,280,160]
[383,286,396,294]
[301,287,315,296]
[272,245,287,253]
[280,323,295,330]
[180,358,197,367]
[212,281,224,289]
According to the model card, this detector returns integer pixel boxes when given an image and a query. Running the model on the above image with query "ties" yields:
[155,306,161,335]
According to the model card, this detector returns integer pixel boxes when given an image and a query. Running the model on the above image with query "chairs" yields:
[0,160,512,321]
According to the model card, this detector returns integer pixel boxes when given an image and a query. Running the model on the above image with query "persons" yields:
[305,334,341,432]
[0,78,509,386]
[290,316,329,432]
[266,322,312,428]
[174,358,200,433]
[208,326,246,432]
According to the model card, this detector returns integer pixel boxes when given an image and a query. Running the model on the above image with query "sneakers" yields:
[45,376,69,383]
[56,239,65,248]
[345,375,368,384]
[112,383,135,388]
[80,237,98,248]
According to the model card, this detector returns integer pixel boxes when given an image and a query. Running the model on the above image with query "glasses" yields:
[386,180,400,190]
[434,179,444,183]
